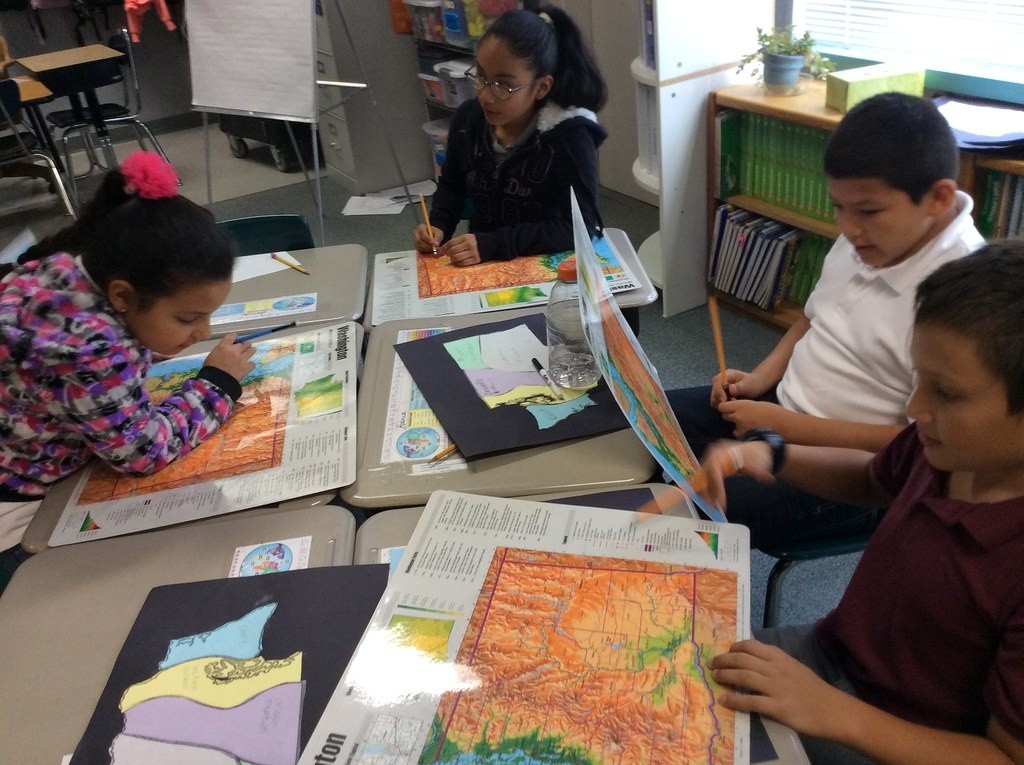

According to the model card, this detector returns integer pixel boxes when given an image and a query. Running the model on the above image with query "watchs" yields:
[739,426,787,475]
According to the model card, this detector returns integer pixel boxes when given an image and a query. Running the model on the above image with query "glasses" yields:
[464,60,543,100]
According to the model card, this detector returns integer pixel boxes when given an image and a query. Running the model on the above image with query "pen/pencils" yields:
[271,253,310,275]
[428,444,457,464]
[233,321,297,344]
[633,447,745,521]
[532,358,563,399]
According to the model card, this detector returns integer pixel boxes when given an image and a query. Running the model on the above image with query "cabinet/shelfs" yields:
[629,1,775,318]
[312,0,438,197]
[411,0,543,183]
[706,76,1024,335]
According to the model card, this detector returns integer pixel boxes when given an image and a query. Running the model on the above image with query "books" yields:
[708,204,832,312]
[714,108,836,227]
[973,169,1024,246]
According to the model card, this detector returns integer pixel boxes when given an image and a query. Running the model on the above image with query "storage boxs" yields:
[826,62,925,111]
[401,0,523,179]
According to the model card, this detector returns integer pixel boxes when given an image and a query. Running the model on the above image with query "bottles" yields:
[545,259,602,388]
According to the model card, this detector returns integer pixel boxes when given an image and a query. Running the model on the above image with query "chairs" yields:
[0,79,79,221]
[46,26,183,203]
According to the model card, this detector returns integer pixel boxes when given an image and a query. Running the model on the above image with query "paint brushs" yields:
[707,298,730,401]
[419,193,438,259]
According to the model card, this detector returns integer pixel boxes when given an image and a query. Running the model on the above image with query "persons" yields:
[640,92,990,558]
[706,237,1024,765]
[414,3,641,340]
[0,152,256,597]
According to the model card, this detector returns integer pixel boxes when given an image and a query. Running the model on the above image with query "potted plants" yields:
[735,23,835,94]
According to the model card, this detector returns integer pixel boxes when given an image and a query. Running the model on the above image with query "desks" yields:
[7,75,68,193]
[0,228,810,765]
[17,43,126,172]
